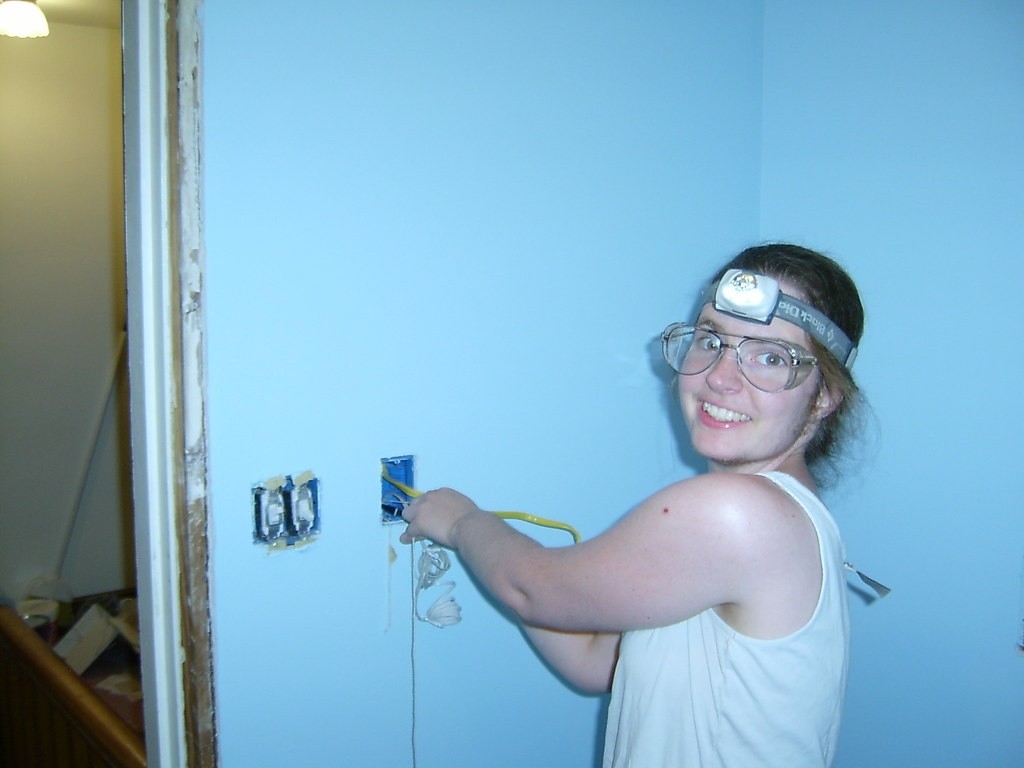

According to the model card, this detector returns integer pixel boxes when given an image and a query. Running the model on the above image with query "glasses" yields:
[662,322,818,393]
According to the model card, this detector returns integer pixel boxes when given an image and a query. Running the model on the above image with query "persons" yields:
[399,244,862,768]
